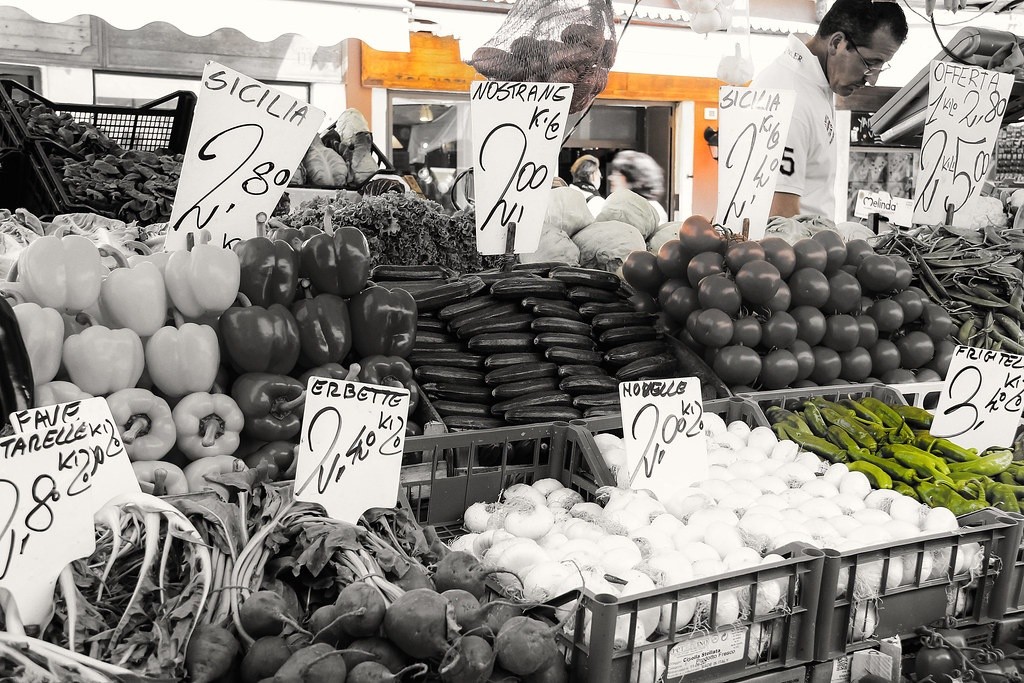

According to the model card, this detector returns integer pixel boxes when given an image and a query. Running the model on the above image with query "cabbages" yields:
[517,184,685,266]
[288,107,409,195]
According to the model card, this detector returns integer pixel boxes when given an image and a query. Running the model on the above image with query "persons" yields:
[606,152,668,224]
[568,155,605,218]
[750,0,908,217]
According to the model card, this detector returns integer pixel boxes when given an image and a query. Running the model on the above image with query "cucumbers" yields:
[374,261,680,432]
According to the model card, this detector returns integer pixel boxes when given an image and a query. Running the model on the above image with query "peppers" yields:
[766,396,1023,514]
[3,204,417,499]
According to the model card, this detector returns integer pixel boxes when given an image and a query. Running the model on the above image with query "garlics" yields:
[444,409,980,650]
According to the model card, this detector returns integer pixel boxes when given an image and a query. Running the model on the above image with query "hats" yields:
[611,150,665,199]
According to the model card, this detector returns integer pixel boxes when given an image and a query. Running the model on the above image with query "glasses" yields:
[843,32,891,75]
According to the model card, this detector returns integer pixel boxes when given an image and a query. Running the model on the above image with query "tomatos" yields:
[624,218,957,394]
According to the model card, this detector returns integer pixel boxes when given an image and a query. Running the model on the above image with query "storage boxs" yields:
[327,378,1023,683]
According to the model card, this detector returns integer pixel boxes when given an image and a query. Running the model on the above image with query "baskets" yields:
[402,378,1022,682]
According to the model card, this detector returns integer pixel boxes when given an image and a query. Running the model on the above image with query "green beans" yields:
[868,222,1022,356]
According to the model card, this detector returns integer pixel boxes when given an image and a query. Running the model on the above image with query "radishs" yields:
[0,501,586,683]
